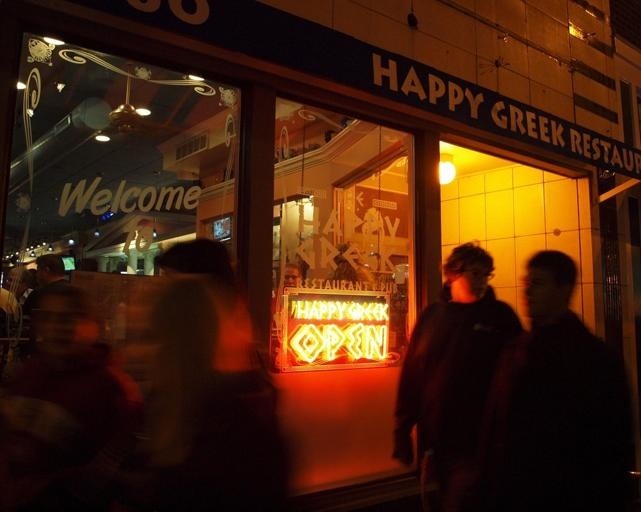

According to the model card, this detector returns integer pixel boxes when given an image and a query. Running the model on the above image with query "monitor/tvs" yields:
[212,216,233,242]
[59,254,77,272]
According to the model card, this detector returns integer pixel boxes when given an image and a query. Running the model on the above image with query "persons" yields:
[389,238,527,512]
[478,248,637,510]
[1,236,295,512]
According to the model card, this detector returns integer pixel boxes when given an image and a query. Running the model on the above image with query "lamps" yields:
[438,152,455,185]
